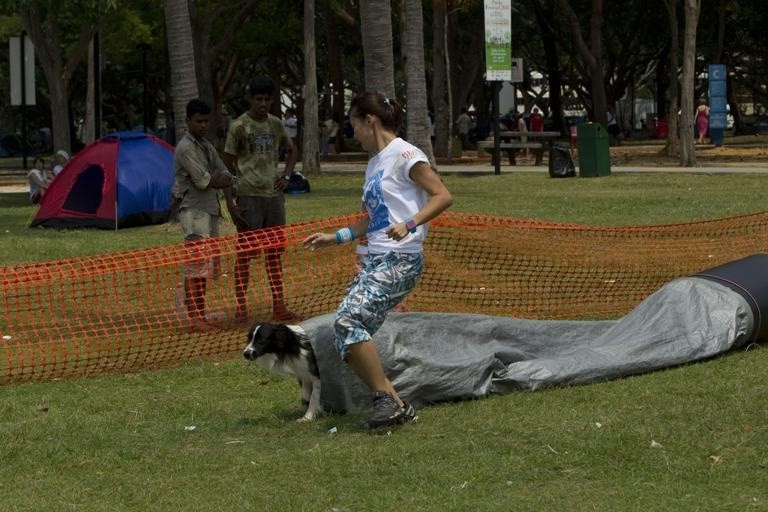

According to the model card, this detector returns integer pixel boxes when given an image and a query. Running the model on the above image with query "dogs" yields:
[242,322,323,425]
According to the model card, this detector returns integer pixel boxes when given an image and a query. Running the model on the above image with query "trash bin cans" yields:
[577,122,611,177]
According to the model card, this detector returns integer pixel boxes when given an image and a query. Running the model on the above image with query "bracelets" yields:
[335,226,355,245]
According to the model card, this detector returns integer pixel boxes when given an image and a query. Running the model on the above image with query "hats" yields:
[56,150,69,162]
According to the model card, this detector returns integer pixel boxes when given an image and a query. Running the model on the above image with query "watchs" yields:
[404,217,417,234]
[282,176,293,181]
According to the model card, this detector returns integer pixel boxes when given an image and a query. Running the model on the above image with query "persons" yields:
[300,91,455,434]
[693,97,710,144]
[27,157,51,205]
[52,150,70,177]
[222,74,301,324]
[169,97,234,335]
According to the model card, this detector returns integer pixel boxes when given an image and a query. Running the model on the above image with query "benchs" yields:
[479,126,564,170]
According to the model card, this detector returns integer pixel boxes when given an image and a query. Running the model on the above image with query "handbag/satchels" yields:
[283,171,310,194]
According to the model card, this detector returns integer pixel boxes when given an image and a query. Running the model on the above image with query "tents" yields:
[24,129,177,231]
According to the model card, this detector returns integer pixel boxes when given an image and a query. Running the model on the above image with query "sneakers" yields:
[360,391,418,431]
